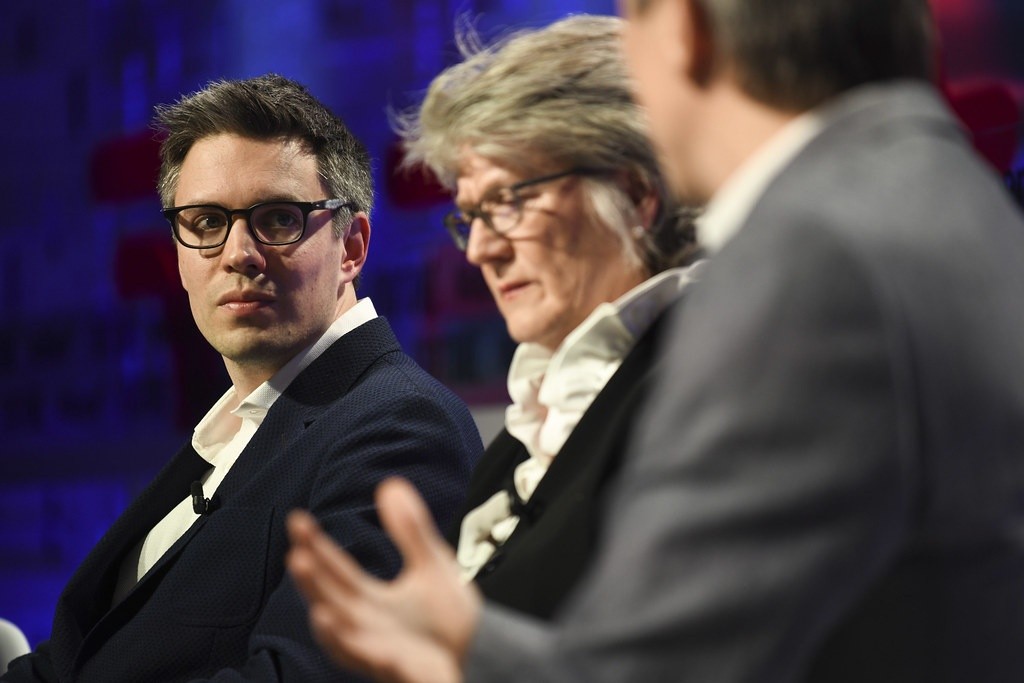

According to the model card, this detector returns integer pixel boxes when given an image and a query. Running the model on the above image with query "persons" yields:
[2,77,482,683]
[286,0,1024,683]
[385,11,711,628]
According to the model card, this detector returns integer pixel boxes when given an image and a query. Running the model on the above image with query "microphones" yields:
[190,480,211,513]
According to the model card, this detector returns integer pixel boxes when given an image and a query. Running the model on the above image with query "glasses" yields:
[157,195,358,250]
[446,167,621,249]
